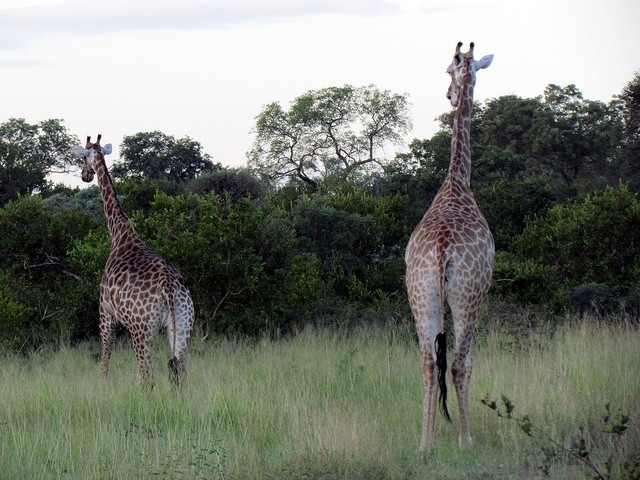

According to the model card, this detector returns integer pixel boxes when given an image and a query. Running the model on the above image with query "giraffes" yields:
[71,133,196,398]
[405,41,495,455]
